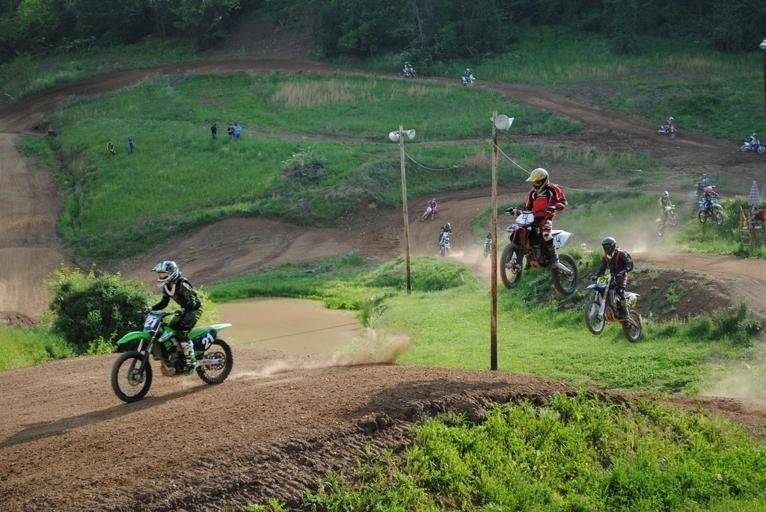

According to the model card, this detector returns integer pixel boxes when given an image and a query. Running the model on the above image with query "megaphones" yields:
[389,130,400,141]
[489,114,514,131]
[403,128,415,139]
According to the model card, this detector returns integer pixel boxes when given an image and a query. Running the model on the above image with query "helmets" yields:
[526,168,549,192]
[602,238,617,260]
[152,260,180,287]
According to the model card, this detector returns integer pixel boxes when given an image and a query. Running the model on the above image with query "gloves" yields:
[546,205,556,212]
[616,273,622,278]
[506,207,514,215]
[591,274,598,281]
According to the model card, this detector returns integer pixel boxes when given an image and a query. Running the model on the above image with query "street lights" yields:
[489,108,516,370]
[389,123,413,295]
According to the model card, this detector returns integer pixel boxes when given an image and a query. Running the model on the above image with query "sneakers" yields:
[183,365,194,374]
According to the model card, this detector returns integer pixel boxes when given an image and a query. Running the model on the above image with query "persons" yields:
[105,139,116,155]
[505,167,568,272]
[127,135,135,154]
[414,195,493,262]
[665,117,674,135]
[591,236,634,321]
[748,133,760,149]
[138,261,202,376]
[462,69,473,86]
[657,172,720,227]
[211,121,241,139]
[403,62,415,77]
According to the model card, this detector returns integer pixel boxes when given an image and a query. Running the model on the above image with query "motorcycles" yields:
[695,183,719,197]
[656,124,679,139]
[699,199,725,226]
[500,205,579,298]
[419,206,432,220]
[739,139,765,156]
[111,308,235,403]
[402,67,416,77]
[440,231,451,258]
[581,271,644,342]
[461,75,477,88]
[484,241,494,258]
[655,203,679,238]
